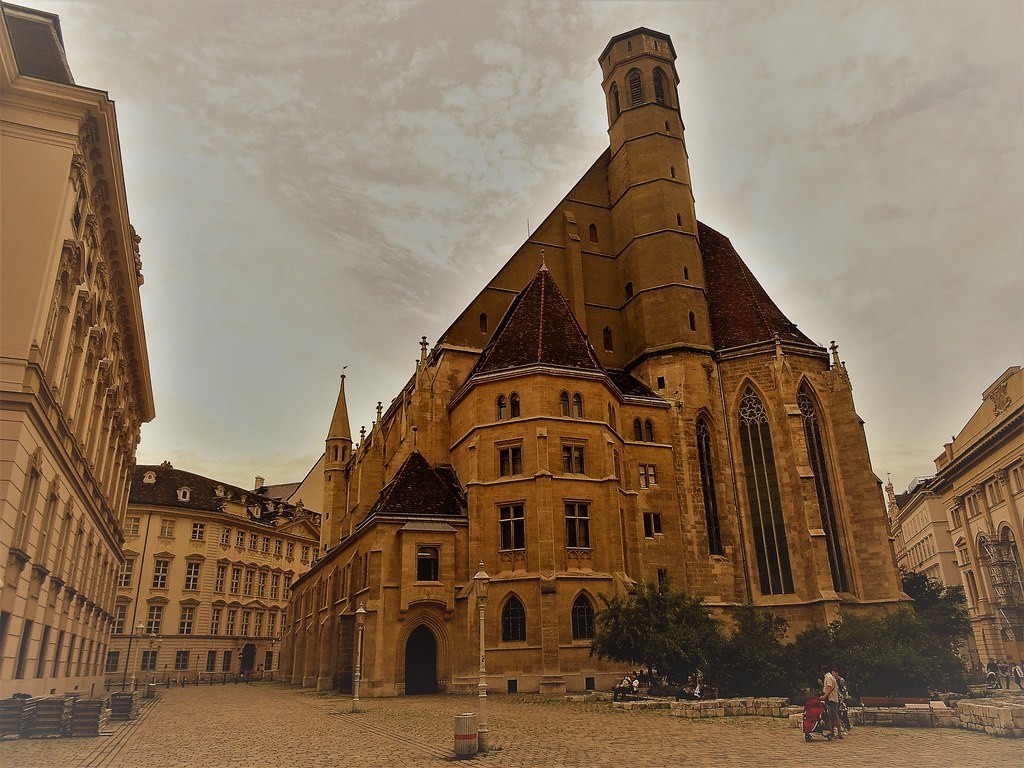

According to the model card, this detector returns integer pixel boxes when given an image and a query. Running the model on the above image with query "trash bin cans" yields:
[454,713,479,759]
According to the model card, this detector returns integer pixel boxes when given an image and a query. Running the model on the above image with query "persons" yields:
[987,658,1024,690]
[818,665,851,739]
[613,670,700,703]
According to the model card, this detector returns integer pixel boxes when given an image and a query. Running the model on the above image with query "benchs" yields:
[861,697,935,728]
[674,685,719,702]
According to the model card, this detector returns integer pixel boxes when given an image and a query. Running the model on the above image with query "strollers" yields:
[801,697,831,742]
[985,671,999,690]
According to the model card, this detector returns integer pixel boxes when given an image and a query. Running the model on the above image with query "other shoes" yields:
[824,734,835,738]
[833,735,843,739]
[843,730,852,736]
[849,725,853,729]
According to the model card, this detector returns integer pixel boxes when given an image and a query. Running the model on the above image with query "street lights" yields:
[130,619,145,692]
[142,630,156,698]
[472,558,492,754]
[350,601,368,713]
[154,636,163,684]
